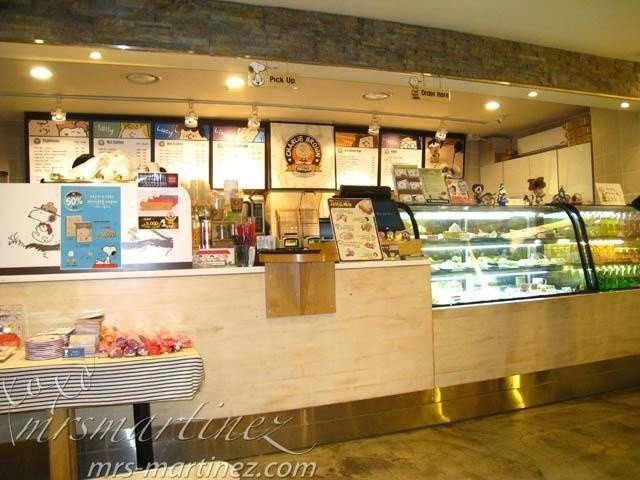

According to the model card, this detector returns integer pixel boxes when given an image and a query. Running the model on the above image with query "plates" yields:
[24,334,66,362]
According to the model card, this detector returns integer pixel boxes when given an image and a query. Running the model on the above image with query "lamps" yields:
[51,94,450,144]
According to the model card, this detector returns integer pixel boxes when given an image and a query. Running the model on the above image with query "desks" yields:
[1,331,204,480]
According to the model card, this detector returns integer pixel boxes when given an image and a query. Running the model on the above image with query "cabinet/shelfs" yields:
[548,202,640,292]
[395,202,597,306]
